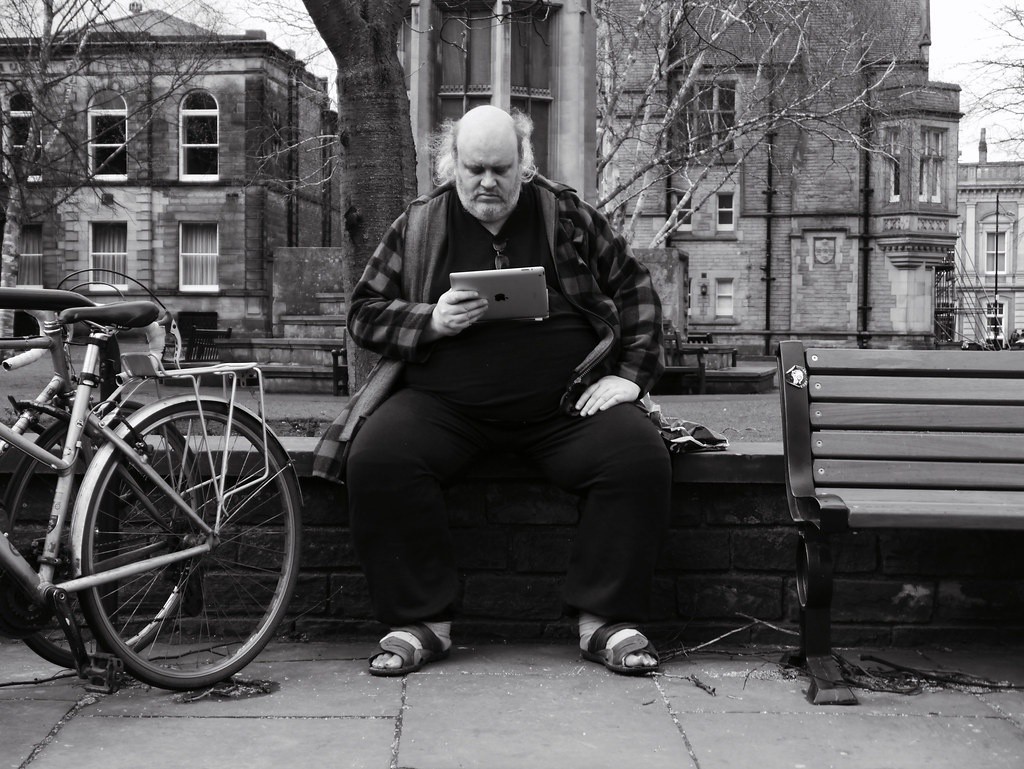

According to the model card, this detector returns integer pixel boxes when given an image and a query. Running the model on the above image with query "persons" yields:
[310,105,730,678]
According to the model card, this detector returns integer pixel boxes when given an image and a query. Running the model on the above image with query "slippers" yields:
[369,621,450,676]
[581,620,659,675]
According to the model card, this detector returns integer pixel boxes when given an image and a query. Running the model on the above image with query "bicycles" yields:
[0,268,308,694]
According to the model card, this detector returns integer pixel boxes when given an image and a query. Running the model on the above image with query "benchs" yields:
[659,330,715,396]
[332,328,349,397]
[773,340,1024,704]
[161,325,232,363]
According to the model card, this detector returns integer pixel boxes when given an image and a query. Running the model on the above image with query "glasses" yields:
[493,236,509,270]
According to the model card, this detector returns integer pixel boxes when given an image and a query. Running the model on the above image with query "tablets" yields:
[449,266,549,320]
